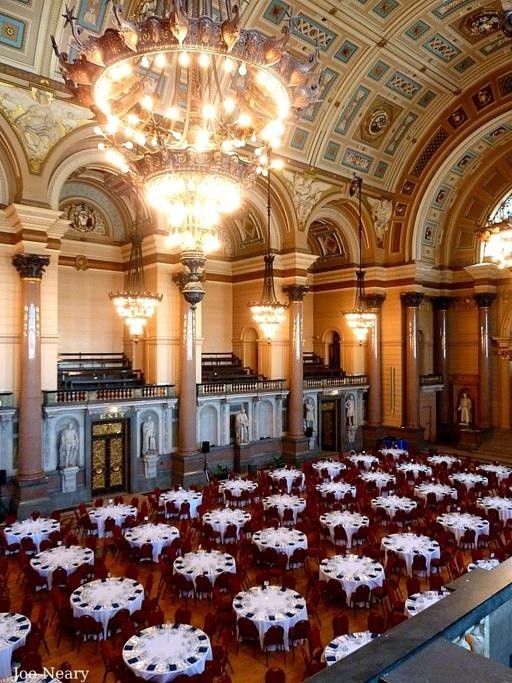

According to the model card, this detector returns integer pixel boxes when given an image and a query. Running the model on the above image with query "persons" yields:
[58,421,80,468]
[344,394,355,425]
[234,408,250,443]
[456,391,472,424]
[304,398,316,432]
[140,415,156,456]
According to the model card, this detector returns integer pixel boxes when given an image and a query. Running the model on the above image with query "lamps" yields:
[45,2,324,312]
[107,196,164,345]
[342,178,378,345]
[477,214,512,276]
[246,150,292,344]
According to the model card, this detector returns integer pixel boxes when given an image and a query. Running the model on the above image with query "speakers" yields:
[306,427,314,438]
[202,441,210,453]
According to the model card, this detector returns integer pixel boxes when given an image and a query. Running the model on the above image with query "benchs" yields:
[303,352,345,379]
[58,353,145,390]
[201,352,258,384]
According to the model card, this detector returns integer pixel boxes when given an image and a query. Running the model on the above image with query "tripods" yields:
[204,453,210,482]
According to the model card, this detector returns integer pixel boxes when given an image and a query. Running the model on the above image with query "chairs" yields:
[0,439,512,683]
